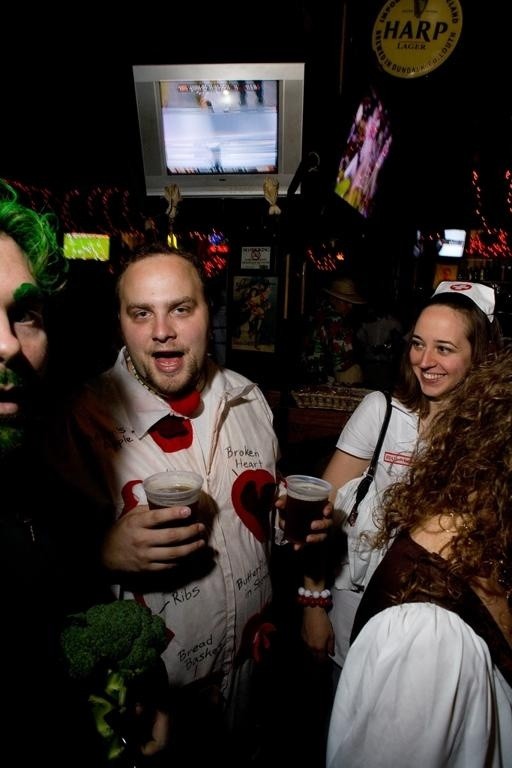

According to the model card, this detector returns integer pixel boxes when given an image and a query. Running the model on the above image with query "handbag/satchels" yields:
[335,478,389,591]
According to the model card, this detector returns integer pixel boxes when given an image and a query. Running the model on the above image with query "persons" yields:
[2,181,512,768]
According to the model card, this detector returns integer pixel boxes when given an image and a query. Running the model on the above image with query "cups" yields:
[145,472,204,552]
[283,474,332,546]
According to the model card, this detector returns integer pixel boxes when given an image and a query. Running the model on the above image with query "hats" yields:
[431,281,495,325]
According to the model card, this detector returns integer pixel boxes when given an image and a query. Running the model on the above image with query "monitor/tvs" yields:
[133,62,305,200]
